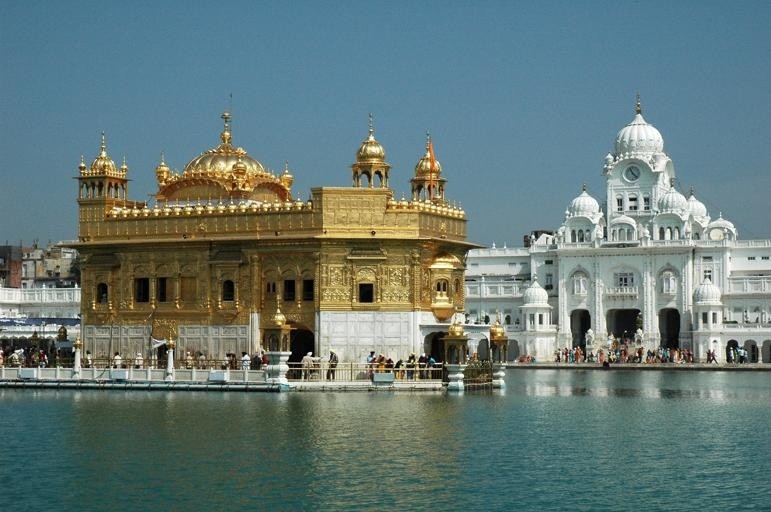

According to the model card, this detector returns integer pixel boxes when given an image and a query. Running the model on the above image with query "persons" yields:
[556,328,694,364]
[728,347,749,364]
[86,350,92,367]
[113,352,122,368]
[327,349,338,382]
[186,350,268,370]
[706,349,718,364]
[0,348,48,368]
[135,353,144,368]
[367,351,436,380]
[301,352,313,382]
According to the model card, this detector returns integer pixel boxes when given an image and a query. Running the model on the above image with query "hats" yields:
[370,351,426,363]
